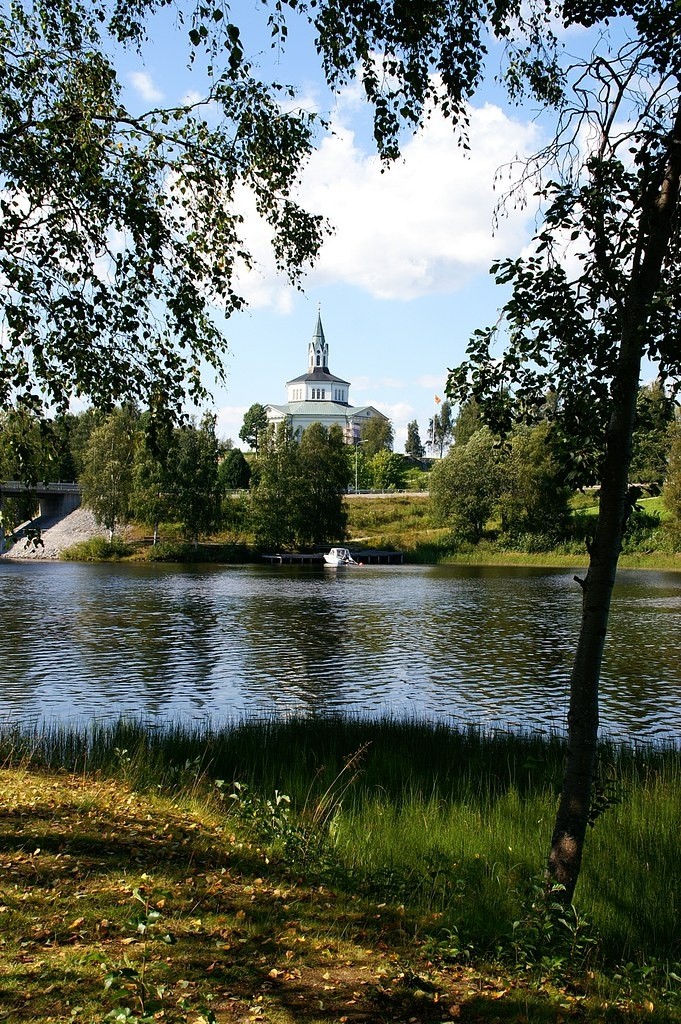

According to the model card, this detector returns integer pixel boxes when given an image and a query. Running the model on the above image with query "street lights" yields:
[356,439,369,495]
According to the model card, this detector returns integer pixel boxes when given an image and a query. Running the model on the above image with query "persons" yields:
[343,554,349,564]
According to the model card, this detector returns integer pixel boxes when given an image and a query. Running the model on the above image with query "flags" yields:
[435,395,441,404]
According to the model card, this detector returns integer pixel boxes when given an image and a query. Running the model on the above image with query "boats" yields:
[324,547,358,566]
